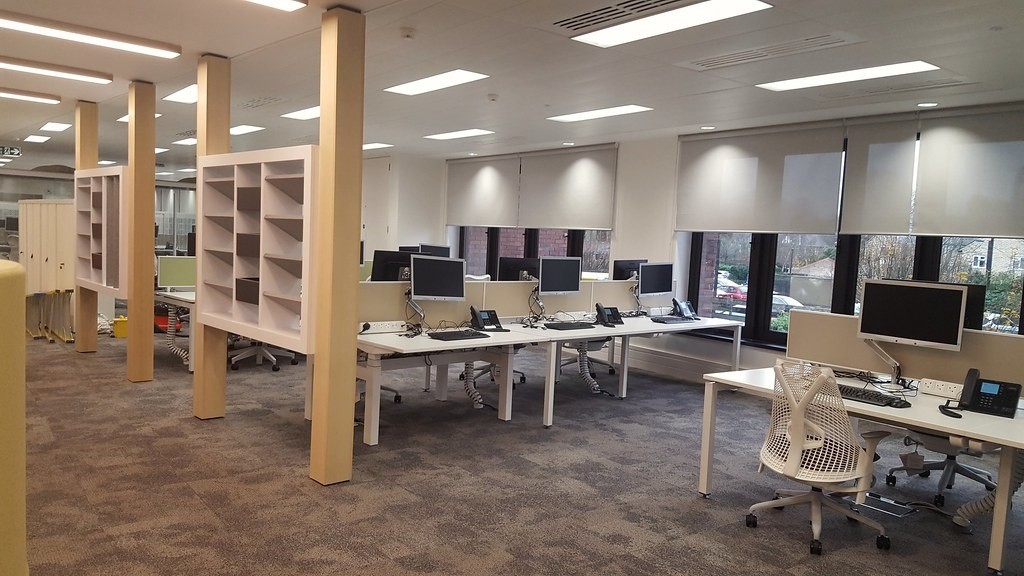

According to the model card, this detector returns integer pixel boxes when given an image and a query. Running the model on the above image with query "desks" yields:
[699,363,1024,571]
[356,308,746,445]
[156,291,195,373]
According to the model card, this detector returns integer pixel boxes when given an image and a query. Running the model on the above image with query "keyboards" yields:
[544,321,595,330]
[425,329,491,341]
[651,316,695,324]
[804,382,901,407]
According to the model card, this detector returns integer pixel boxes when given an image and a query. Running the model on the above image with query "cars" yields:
[983,313,1020,333]
[716,278,748,303]
[772,295,803,312]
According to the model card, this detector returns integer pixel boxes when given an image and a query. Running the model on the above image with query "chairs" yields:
[747,358,891,555]
[561,337,617,378]
[887,429,998,505]
[459,344,528,390]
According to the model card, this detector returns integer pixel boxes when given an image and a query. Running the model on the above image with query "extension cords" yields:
[918,378,965,400]
[557,311,589,321]
[648,307,673,317]
[359,321,407,334]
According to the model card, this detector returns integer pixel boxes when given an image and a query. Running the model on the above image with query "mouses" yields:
[604,323,616,328]
[890,399,912,408]
[681,316,694,320]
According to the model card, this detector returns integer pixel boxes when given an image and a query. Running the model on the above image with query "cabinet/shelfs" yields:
[197,144,315,355]
[74,165,128,302]
[17,199,115,333]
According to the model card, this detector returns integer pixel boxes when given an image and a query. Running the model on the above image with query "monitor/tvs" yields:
[497,256,582,295]
[613,260,674,298]
[857,278,987,351]
[371,244,466,302]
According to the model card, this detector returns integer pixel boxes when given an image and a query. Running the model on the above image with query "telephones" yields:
[672,297,701,321]
[593,302,624,327]
[469,305,511,332]
[938,368,1022,419]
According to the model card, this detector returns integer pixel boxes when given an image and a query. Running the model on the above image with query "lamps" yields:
[244,0,309,13]
[0,87,61,105]
[0,56,113,85]
[0,10,181,59]
